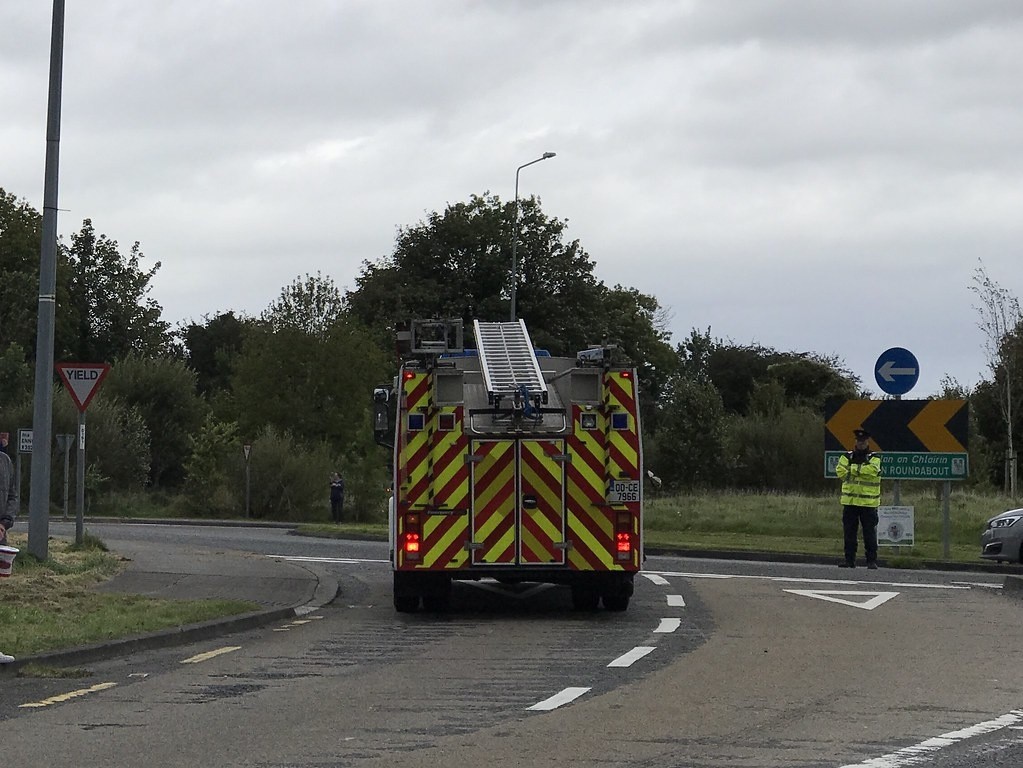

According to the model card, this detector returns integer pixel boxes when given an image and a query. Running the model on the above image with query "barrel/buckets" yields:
[0,544,20,577]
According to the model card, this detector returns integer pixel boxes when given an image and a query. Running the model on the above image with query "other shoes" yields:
[867,560,878,569]
[838,560,856,568]
[0,652,15,663]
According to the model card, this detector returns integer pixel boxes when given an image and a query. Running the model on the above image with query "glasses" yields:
[856,438,867,442]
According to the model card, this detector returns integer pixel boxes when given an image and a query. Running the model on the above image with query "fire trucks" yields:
[372,316,662,613]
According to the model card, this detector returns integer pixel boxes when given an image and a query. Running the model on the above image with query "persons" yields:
[330,472,345,525]
[836,430,882,570]
[0,451,16,546]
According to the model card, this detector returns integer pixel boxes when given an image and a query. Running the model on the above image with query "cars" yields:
[980,508,1023,568]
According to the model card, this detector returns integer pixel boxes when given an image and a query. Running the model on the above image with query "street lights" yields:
[511,153,556,321]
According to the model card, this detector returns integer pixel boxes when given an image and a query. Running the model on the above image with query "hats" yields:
[853,430,872,438]
